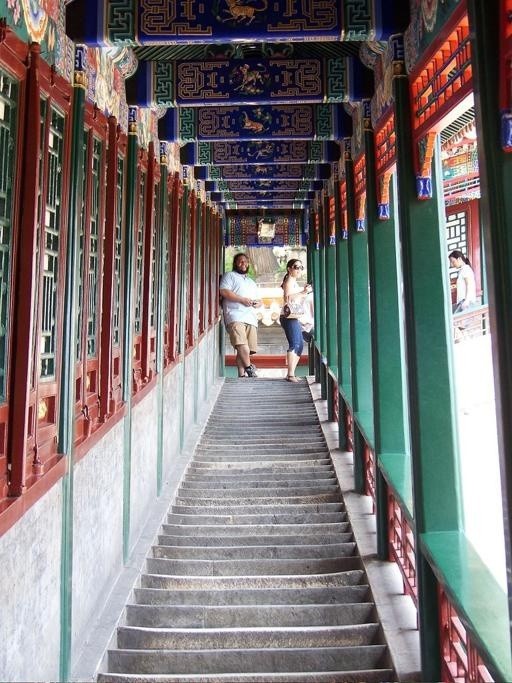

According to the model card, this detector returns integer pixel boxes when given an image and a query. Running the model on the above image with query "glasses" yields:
[293,264,304,270]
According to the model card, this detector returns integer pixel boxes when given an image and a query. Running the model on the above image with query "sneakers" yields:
[244,363,258,377]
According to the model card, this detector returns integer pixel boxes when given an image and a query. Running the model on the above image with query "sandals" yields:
[286,373,303,383]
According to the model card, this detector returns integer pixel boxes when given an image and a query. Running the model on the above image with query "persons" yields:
[219,251,265,377]
[447,249,478,328]
[278,257,315,384]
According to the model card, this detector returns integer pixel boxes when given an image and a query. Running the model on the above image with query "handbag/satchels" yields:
[283,293,305,319]
[301,322,314,343]
